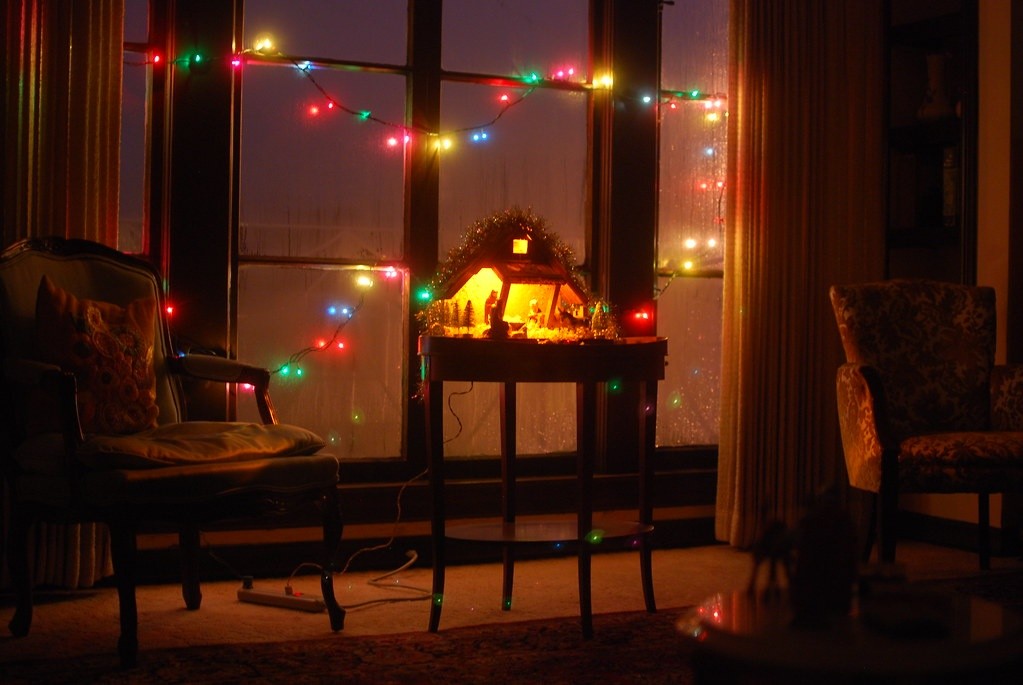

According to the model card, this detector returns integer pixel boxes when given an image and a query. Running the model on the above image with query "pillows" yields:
[29,274,160,431]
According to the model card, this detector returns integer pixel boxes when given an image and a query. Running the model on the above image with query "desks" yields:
[420,333,669,634]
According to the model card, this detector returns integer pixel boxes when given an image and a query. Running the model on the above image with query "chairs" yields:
[0,241,347,647]
[830,281,1023,597]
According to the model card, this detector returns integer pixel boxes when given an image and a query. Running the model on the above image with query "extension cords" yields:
[236,587,326,611]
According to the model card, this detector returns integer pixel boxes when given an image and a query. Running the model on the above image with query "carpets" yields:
[0,569,1023,684]
